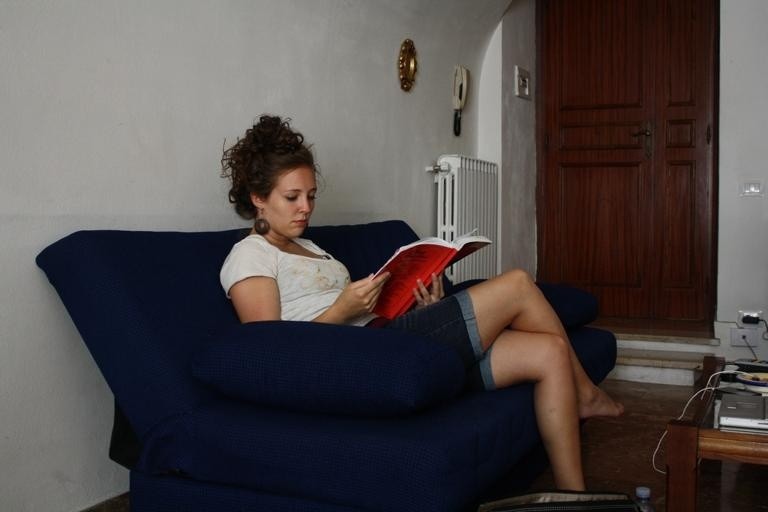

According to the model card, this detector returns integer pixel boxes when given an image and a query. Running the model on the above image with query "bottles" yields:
[635,488,652,511]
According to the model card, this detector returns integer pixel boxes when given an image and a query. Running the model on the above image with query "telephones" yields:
[452,66,470,110]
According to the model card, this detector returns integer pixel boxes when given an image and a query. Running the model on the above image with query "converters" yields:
[742,315,759,324]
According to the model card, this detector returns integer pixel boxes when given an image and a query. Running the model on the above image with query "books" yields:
[367,228,492,320]
[713,392,767,436]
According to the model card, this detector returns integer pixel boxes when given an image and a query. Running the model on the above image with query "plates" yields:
[735,373,768,386]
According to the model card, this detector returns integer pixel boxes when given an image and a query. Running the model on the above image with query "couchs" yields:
[36,219,617,511]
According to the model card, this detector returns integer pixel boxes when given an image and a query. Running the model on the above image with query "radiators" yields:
[424,153,499,285]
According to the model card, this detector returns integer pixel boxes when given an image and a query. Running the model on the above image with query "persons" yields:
[217,114,625,493]
